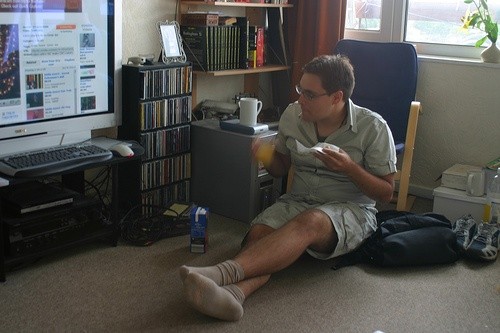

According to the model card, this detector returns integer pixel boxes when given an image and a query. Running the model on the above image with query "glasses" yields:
[295,84,330,102]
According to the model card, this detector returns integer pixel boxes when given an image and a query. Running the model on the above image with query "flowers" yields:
[460,0,499,48]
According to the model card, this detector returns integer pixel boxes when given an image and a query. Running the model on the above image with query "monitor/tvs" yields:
[0,0,124,139]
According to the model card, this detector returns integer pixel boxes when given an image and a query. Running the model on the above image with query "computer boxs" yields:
[190,119,282,223]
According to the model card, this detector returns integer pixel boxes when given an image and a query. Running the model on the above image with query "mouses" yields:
[109,144,136,157]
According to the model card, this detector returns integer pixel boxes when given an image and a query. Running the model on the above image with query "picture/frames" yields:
[156,19,187,64]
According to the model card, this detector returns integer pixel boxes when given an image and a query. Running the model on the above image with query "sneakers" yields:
[465,216,500,262]
[452,213,478,252]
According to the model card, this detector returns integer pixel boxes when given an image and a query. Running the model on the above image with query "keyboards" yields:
[0,143,114,178]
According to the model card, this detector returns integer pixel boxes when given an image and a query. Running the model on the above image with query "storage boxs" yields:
[190,206,209,253]
[436,164,483,192]
[180,12,218,27]
[431,187,500,225]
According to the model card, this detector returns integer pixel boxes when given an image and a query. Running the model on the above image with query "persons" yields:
[175,56,397,321]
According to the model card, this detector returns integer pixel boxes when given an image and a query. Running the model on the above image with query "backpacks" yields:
[330,209,460,272]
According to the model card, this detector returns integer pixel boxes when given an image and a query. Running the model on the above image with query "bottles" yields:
[487,168,500,206]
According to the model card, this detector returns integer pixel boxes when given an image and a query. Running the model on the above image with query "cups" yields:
[239,97,263,126]
[466,170,484,196]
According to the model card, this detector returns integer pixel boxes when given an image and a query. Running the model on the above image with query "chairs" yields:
[286,40,423,212]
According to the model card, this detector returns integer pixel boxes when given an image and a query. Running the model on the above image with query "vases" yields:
[480,42,500,63]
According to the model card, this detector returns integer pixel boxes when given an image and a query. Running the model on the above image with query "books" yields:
[181,0,290,69]
[141,65,192,220]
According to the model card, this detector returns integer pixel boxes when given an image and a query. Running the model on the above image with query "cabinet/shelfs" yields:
[118,61,194,242]
[0,144,143,282]
[179,0,294,128]
